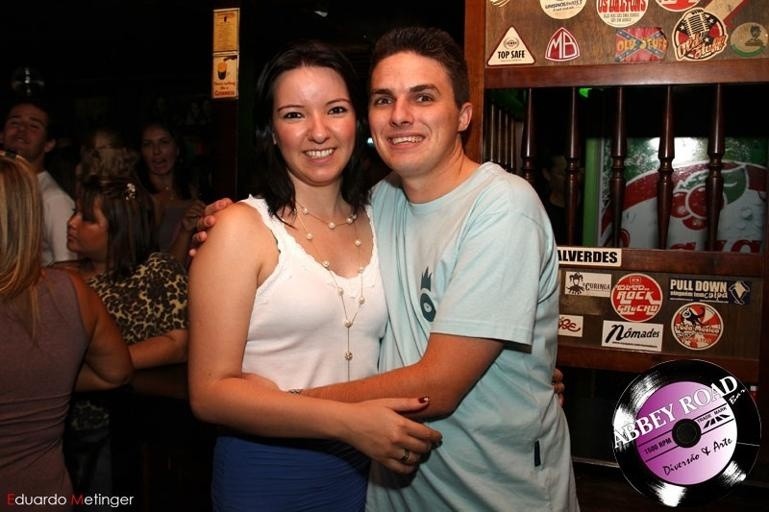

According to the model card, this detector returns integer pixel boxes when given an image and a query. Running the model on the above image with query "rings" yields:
[400,449,409,463]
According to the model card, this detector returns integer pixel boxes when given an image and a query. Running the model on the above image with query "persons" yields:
[0,94,84,268]
[71,127,137,194]
[535,144,571,246]
[0,148,137,511]
[46,172,188,511]
[127,107,203,266]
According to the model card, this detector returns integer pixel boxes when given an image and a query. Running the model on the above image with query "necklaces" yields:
[287,197,366,382]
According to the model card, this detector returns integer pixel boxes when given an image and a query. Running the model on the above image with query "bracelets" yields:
[288,387,304,395]
